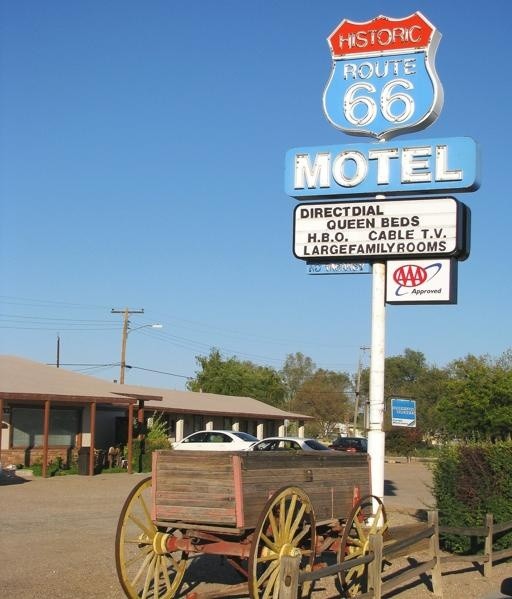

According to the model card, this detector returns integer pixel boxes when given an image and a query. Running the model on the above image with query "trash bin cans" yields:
[78,447,90,475]
[407,456,410,463]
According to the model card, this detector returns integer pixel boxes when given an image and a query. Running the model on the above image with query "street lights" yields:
[118,323,167,384]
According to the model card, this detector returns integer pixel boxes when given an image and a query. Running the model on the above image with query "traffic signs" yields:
[391,399,417,427]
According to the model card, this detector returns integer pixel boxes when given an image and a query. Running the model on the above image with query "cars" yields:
[168,428,369,455]
